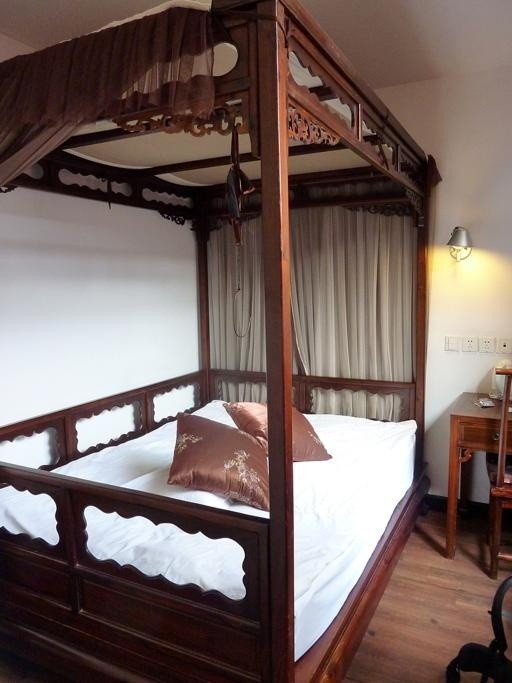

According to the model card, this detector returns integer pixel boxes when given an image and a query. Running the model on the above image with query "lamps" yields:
[446,225,474,262]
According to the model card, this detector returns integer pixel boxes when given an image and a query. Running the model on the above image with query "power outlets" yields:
[478,337,495,354]
[462,335,478,352]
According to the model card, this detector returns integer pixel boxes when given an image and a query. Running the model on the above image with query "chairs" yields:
[446,577,512,682]
[487,367,512,580]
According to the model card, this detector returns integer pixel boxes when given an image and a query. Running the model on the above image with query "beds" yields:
[1,2,440,683]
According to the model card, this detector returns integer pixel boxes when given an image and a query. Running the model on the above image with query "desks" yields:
[446,393,512,559]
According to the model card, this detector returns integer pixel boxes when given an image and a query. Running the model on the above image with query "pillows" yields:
[167,412,272,512]
[221,399,333,461]
